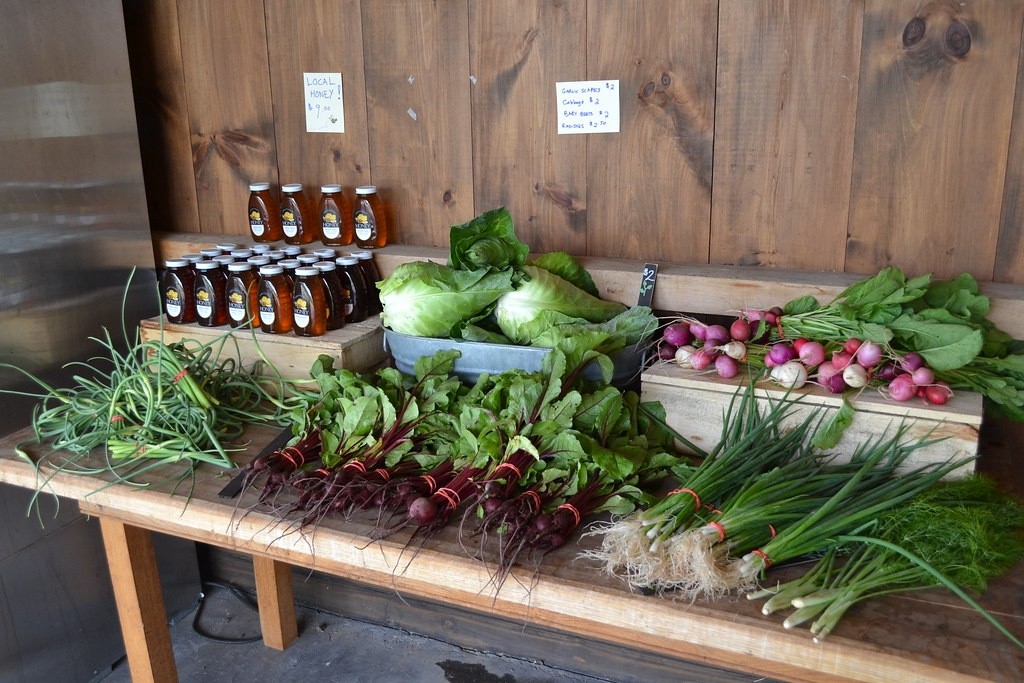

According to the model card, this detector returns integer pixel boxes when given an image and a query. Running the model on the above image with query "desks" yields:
[0,372,1024,681]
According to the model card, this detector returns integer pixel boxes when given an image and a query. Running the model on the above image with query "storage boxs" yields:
[382,316,654,397]
[140,310,393,399]
[640,356,984,483]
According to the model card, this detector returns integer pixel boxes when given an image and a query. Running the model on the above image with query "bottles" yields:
[318,185,354,247]
[280,183,313,244]
[165,243,382,338]
[248,183,282,243]
[353,186,388,249]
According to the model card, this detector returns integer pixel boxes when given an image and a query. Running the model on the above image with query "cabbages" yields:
[374,206,658,348]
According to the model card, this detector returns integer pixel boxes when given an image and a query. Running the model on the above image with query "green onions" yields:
[579,366,1024,651]
